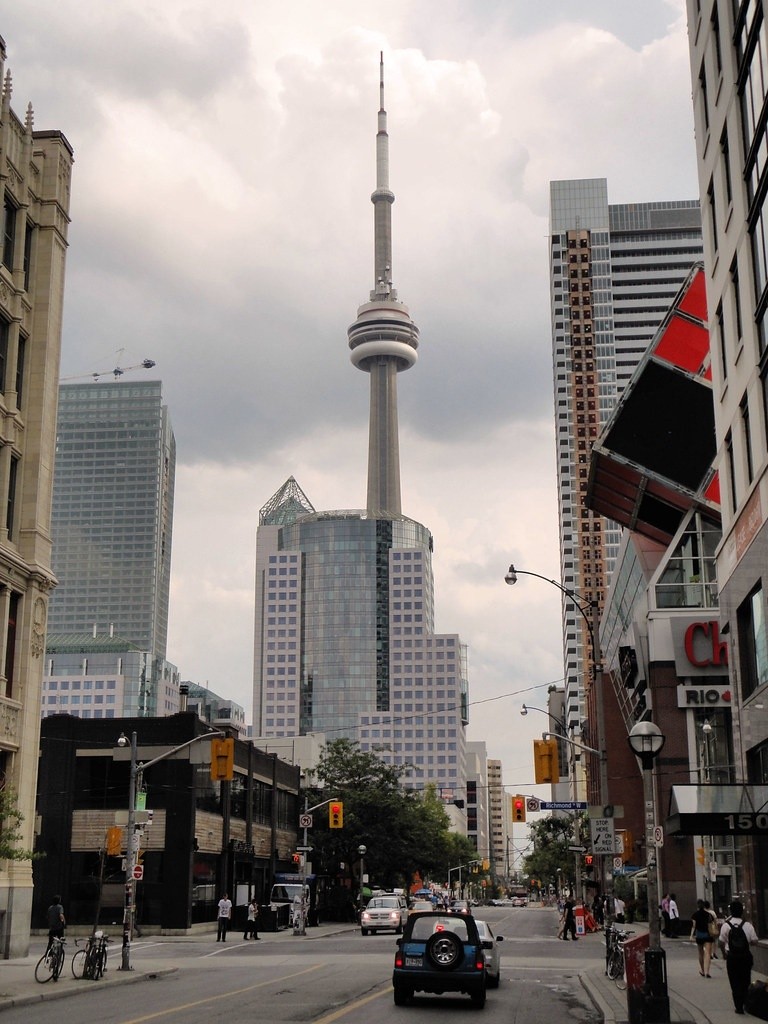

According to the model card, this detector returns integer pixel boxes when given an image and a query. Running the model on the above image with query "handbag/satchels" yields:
[709,922,719,935]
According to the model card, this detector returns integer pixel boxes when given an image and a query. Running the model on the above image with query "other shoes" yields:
[563,937,569,941]
[572,937,578,940]
[557,935,562,940]
[710,956,713,959]
[713,953,718,959]
[734,1008,744,1014]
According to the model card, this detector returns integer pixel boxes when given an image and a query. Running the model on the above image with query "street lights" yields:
[520,703,584,906]
[503,564,618,931]
[626,721,672,1024]
[116,730,139,970]
[357,845,368,926]
[556,868,563,901]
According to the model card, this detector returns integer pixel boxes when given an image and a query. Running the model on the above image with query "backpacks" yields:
[726,920,749,953]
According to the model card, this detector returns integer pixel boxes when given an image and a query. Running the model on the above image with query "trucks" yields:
[267,874,326,928]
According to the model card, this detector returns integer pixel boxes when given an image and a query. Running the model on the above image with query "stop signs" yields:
[132,864,144,880]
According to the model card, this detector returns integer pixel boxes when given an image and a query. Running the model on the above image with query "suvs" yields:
[392,911,494,1010]
[360,896,408,936]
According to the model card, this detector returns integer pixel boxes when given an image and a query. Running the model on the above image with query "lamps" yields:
[702,717,717,734]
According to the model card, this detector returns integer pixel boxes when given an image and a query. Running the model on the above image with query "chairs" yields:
[416,923,433,940]
[453,927,468,941]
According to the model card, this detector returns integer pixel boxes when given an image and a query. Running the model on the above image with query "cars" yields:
[447,900,473,915]
[409,901,433,914]
[433,918,504,989]
[488,895,529,909]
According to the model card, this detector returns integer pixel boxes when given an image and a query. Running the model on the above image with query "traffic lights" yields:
[510,795,526,823]
[531,879,535,885]
[292,853,303,865]
[328,801,343,828]
[584,856,594,867]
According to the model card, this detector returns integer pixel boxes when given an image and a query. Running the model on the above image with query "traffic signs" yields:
[591,818,618,856]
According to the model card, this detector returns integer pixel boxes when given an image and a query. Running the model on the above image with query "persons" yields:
[558,895,579,940]
[123,897,143,937]
[45,895,66,956]
[244,899,260,940]
[433,894,449,912]
[704,901,718,959]
[216,893,232,942]
[690,900,715,978]
[719,900,758,1014]
[405,893,432,907]
[660,893,680,939]
[591,892,625,931]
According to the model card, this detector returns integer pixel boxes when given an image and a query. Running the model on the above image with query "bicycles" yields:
[605,924,636,992]
[33,934,68,984]
[72,934,114,981]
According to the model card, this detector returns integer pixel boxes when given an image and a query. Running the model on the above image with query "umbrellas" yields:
[415,889,432,897]
